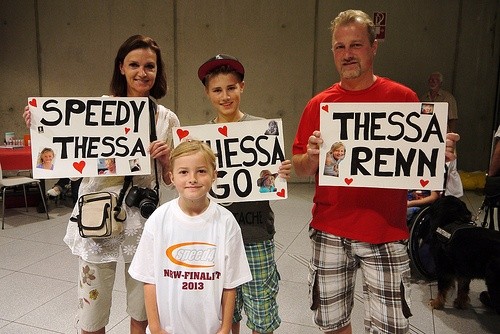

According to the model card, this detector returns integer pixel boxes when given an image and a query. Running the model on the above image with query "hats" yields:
[198,53,245,83]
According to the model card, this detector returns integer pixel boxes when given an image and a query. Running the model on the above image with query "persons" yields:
[36,148,54,170]
[198,52,294,334]
[128,141,253,334]
[291,10,464,334]
[45,33,180,334]
[99,158,115,174]
[488,125,500,177]
[257,170,278,192]
[265,121,279,135]
[131,159,142,172]
[324,142,346,177]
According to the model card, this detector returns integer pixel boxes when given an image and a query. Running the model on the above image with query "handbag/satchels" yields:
[70,190,127,241]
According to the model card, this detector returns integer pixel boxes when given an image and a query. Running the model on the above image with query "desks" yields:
[0,141,32,208]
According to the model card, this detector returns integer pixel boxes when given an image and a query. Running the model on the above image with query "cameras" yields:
[125,185,159,218]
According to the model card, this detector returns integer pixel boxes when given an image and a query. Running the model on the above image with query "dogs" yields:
[407,195,499,310]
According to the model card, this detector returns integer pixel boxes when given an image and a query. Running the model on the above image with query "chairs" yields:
[0,162,49,230]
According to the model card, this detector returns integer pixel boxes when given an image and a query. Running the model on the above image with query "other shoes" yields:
[47,189,61,196]
